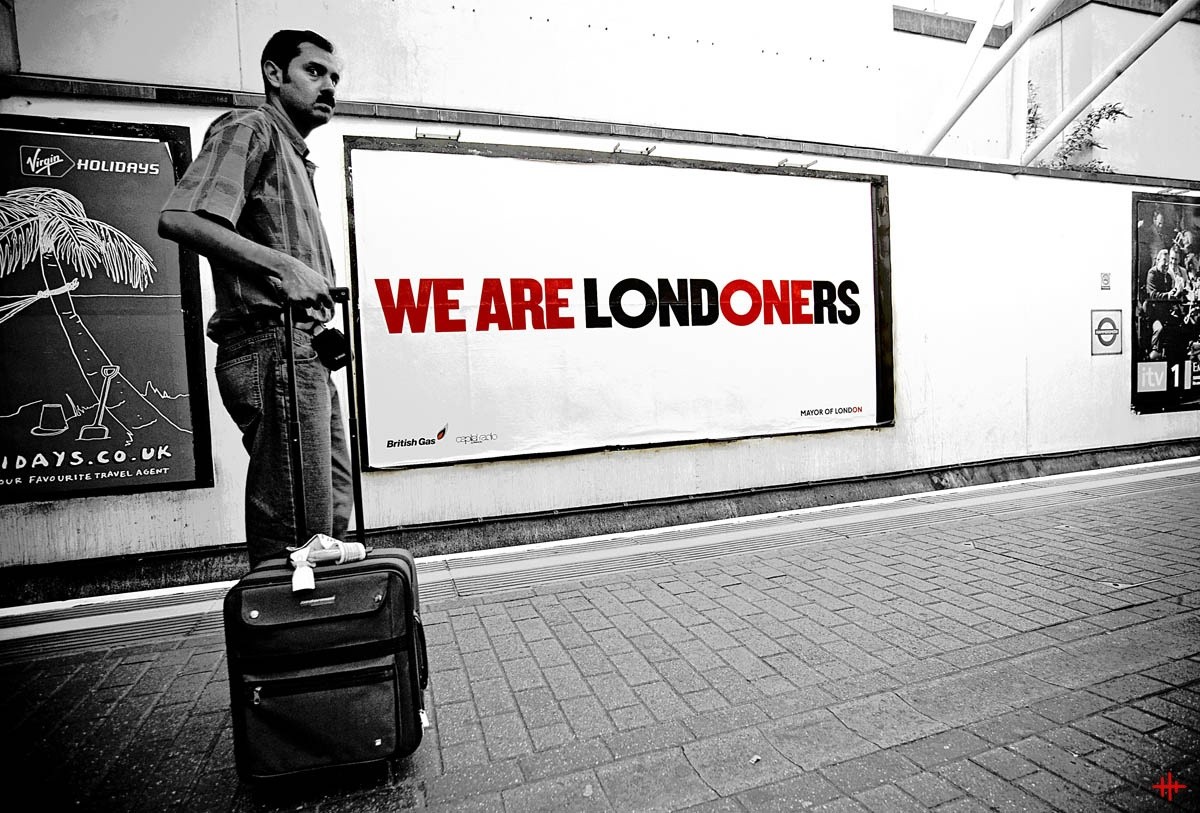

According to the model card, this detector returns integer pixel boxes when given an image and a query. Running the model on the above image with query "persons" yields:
[157,29,358,571]
[1137,209,1200,364]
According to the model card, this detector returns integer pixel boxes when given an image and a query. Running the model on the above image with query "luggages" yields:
[221,287,430,793]
